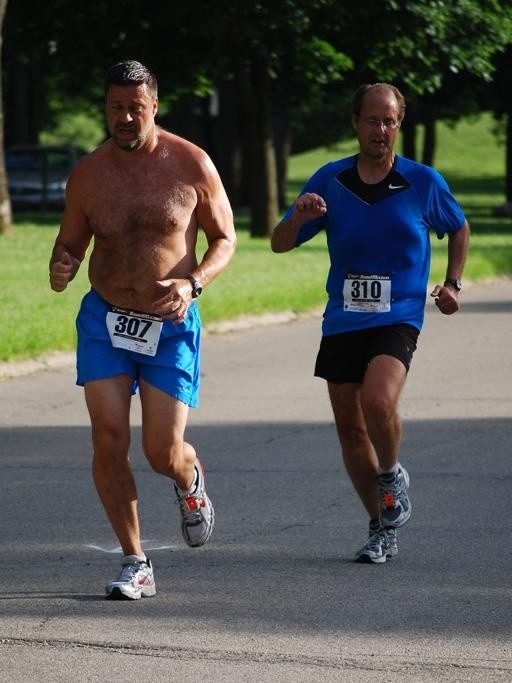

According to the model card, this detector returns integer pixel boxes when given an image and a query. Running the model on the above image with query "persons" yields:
[49,57,240,600]
[271,82,473,566]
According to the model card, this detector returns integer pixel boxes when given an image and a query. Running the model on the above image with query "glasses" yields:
[355,116,397,129]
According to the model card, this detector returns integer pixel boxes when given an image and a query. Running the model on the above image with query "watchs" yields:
[185,273,203,300]
[443,278,463,291]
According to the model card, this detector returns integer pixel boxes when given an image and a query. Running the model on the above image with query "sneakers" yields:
[174,453,215,547]
[355,517,398,564]
[103,553,156,600]
[377,463,412,530]
